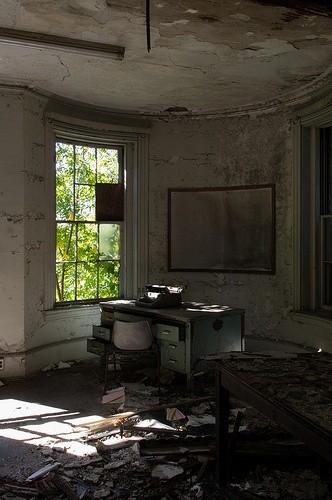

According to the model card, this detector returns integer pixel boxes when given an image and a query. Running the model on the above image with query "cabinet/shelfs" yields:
[86,297,246,387]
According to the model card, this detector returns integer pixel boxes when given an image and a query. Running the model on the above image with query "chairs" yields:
[104,319,161,396]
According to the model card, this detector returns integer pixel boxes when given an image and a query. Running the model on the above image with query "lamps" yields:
[0,27,125,62]
